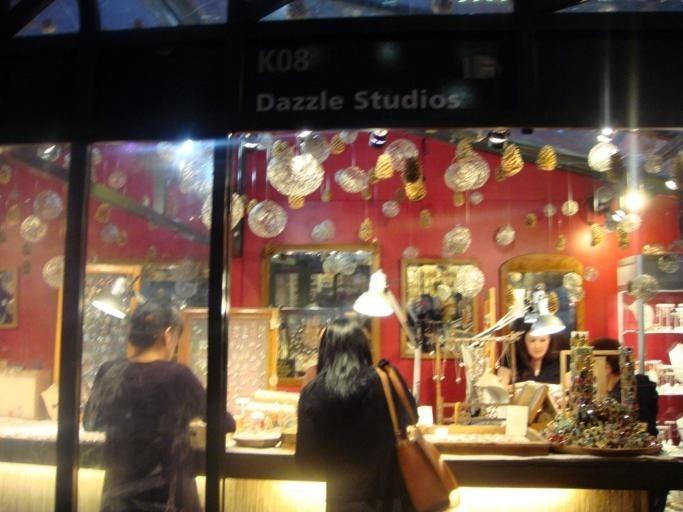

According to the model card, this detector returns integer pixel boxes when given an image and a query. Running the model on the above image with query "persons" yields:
[82,296,236,511]
[587,335,659,438]
[495,326,569,384]
[294,315,420,512]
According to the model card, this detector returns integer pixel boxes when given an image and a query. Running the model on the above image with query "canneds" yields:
[634,302,683,446]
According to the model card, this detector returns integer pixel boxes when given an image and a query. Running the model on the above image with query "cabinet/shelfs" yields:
[616,251,683,450]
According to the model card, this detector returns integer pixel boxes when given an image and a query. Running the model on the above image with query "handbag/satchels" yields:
[395,427,461,512]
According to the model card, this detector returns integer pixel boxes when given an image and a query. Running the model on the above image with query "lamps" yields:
[353,268,568,432]
[91,272,130,320]
